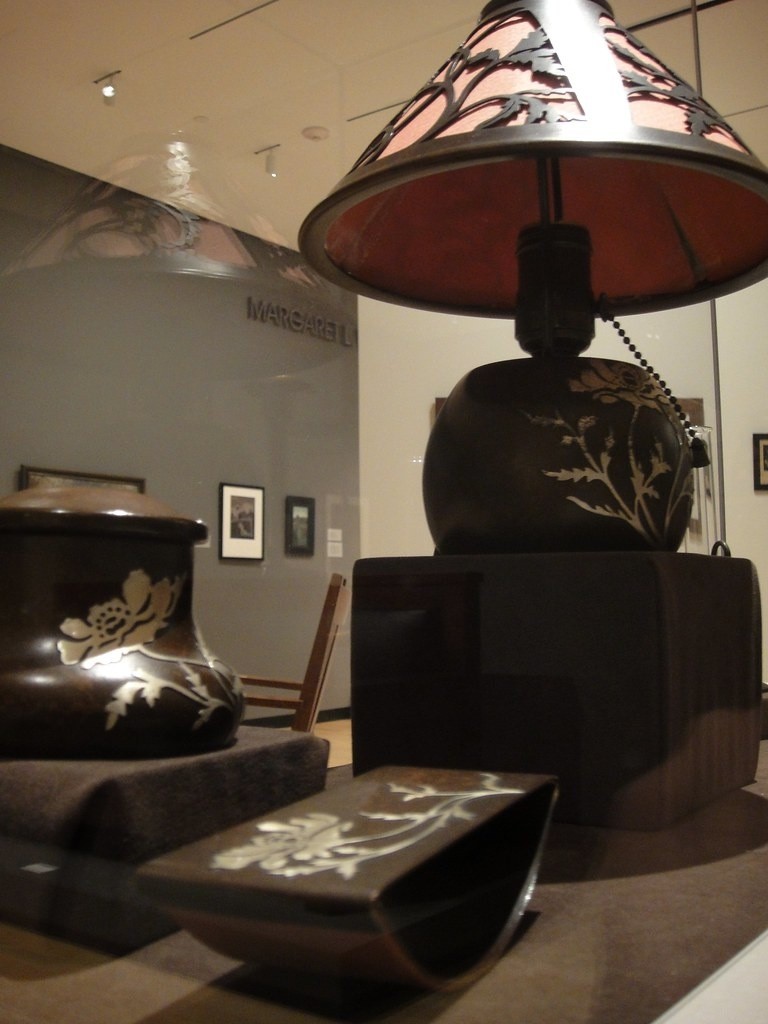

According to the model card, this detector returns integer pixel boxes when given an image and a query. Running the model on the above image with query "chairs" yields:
[234,573,347,733]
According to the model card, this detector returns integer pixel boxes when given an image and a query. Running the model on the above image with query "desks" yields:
[132,765,572,1004]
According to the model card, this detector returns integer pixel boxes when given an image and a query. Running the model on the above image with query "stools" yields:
[0,731,330,956]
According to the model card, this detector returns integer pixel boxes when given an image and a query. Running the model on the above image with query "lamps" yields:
[295,0,768,555]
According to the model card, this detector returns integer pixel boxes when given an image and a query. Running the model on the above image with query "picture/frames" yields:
[752,433,768,490]
[218,481,264,560]
[284,497,316,556]
[19,464,146,496]
